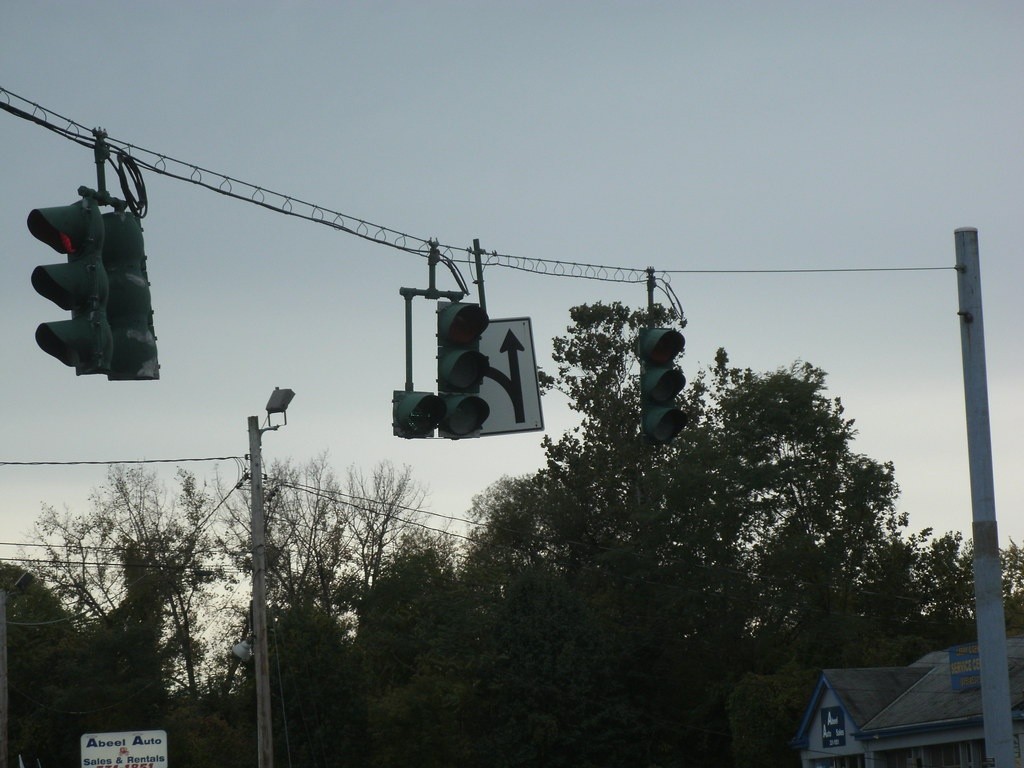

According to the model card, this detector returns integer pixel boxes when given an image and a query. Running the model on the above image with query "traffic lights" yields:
[26,195,115,375]
[638,325,689,446]
[436,301,491,439]
[392,390,449,439]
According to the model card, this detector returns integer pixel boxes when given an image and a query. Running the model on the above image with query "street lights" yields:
[248,387,298,768]
